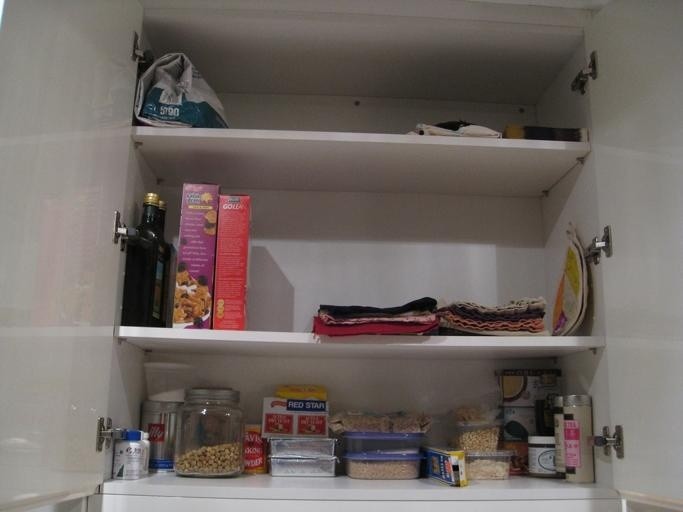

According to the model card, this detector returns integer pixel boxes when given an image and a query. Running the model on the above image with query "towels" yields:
[547,218,589,336]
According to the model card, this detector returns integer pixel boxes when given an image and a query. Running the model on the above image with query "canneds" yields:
[244,423,268,474]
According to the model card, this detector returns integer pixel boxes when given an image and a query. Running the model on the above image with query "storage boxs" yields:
[502,441,529,474]
[463,449,512,480]
[453,419,504,450]
[261,395,329,440]
[495,366,564,407]
[501,406,538,441]
[341,430,427,480]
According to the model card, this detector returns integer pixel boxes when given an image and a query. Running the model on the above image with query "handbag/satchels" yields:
[133,52,230,127]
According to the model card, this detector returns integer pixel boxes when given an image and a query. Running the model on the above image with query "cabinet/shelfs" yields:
[1,1,682,512]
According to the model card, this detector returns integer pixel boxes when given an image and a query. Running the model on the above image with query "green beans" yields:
[447,430,498,453]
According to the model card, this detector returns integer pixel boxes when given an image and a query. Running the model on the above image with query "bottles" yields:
[120,192,172,327]
[111,430,150,480]
[535,372,593,484]
[172,388,245,477]
